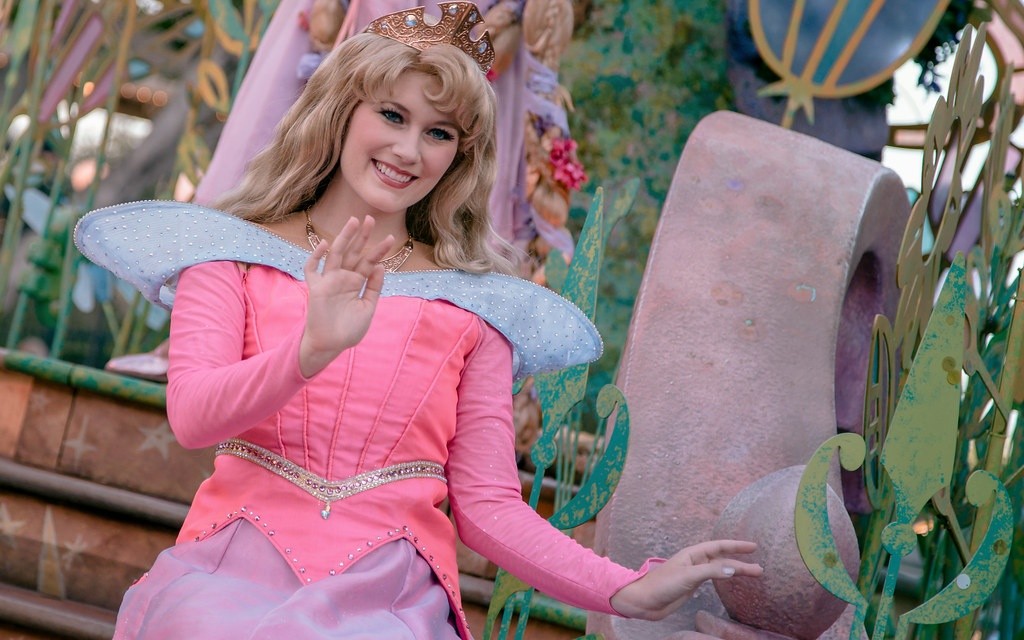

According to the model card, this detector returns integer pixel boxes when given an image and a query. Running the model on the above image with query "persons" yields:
[112,34,763,640]
[105,0,527,385]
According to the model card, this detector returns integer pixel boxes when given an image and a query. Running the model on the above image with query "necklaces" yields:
[306,204,414,273]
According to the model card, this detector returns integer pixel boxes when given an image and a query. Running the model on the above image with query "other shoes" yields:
[103,337,169,383]
